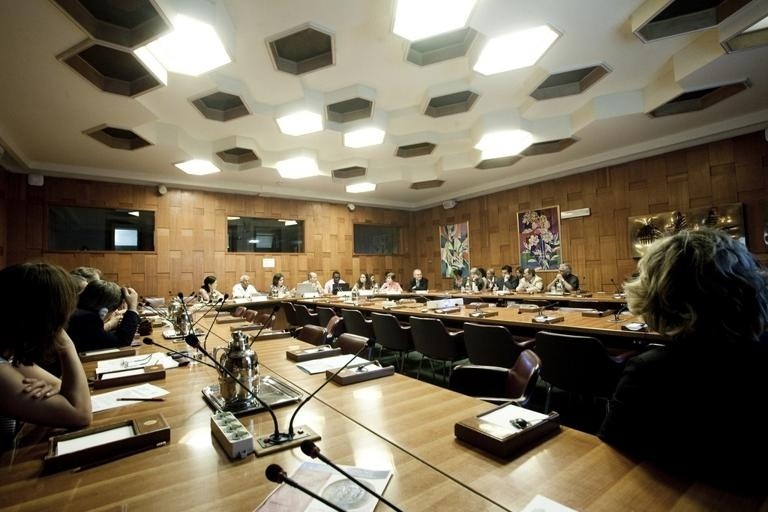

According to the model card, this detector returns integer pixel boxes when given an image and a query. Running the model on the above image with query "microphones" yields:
[137,285,402,512]
[412,275,628,324]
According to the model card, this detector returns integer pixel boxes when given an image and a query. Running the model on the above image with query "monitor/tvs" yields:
[110,225,141,250]
[255,233,277,251]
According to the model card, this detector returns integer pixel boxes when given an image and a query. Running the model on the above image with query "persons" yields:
[194,268,429,303]
[450,262,579,294]
[595,228,768,498]
[0,262,139,431]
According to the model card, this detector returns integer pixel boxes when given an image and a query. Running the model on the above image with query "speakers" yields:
[29,174,44,186]
[444,200,456,211]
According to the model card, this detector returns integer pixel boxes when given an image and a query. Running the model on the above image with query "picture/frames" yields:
[516,205,563,273]
[438,220,471,280]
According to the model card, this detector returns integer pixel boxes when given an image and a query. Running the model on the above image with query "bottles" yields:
[211,330,260,405]
[167,295,191,338]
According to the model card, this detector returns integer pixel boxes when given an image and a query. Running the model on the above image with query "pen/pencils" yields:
[116,398,165,401]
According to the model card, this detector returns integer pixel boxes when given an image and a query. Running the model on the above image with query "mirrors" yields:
[224,215,306,256]
[353,222,409,257]
[41,203,159,255]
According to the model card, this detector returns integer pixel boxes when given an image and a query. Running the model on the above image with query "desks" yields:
[0,289,768,512]
